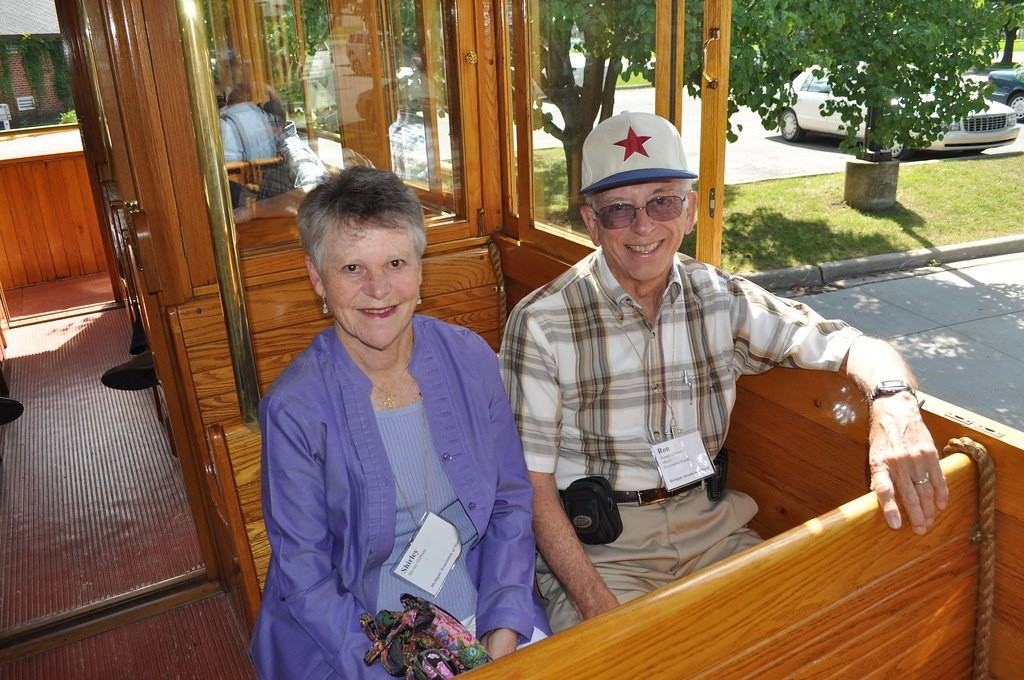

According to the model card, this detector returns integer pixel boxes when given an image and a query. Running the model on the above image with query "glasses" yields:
[590,194,688,229]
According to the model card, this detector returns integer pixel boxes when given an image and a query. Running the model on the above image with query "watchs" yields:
[868,380,925,408]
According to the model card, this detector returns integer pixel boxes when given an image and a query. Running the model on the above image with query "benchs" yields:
[159,189,1000,680]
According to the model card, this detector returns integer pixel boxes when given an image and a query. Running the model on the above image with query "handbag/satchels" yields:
[359,593,493,680]
[563,476,624,546]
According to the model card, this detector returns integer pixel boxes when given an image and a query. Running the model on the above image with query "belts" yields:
[556,479,711,505]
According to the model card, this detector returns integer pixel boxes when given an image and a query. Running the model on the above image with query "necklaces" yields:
[372,358,409,408]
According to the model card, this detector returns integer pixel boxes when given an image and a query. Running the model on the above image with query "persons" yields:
[101,56,328,390]
[499,110,949,636]
[249,166,553,680]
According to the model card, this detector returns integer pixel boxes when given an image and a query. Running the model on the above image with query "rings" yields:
[914,478,928,485]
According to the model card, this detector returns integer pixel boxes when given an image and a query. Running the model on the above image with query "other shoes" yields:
[101,351,159,391]
[1,395,23,427]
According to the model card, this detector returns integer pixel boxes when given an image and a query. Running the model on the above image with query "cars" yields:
[983,65,1024,123]
[759,61,1020,160]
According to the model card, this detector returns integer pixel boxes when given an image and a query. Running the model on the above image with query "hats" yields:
[580,110,698,194]
